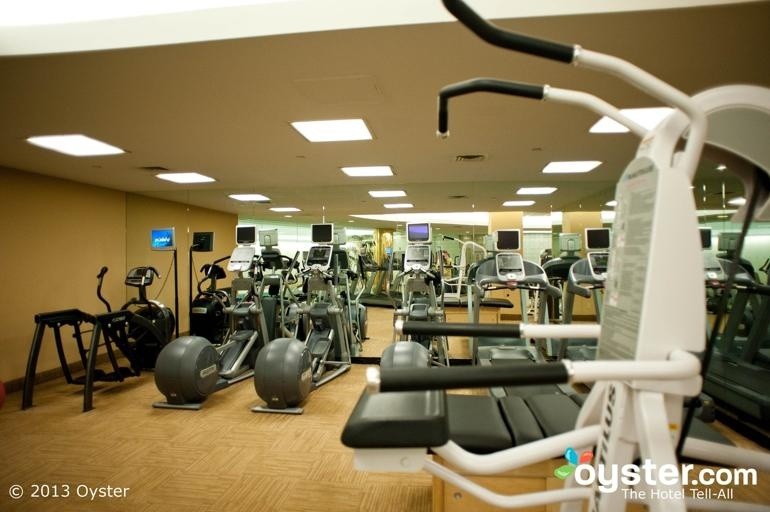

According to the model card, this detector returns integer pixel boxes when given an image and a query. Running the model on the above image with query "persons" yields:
[435,250,454,279]
[539,249,563,324]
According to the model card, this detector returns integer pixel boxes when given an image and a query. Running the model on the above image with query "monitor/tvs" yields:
[718,233,743,251]
[384,247,392,255]
[494,229,521,252]
[310,223,333,245]
[151,228,176,251]
[235,225,257,245]
[585,228,612,250]
[192,232,214,251]
[259,229,278,246]
[698,226,712,250]
[558,233,581,251]
[405,222,431,245]
